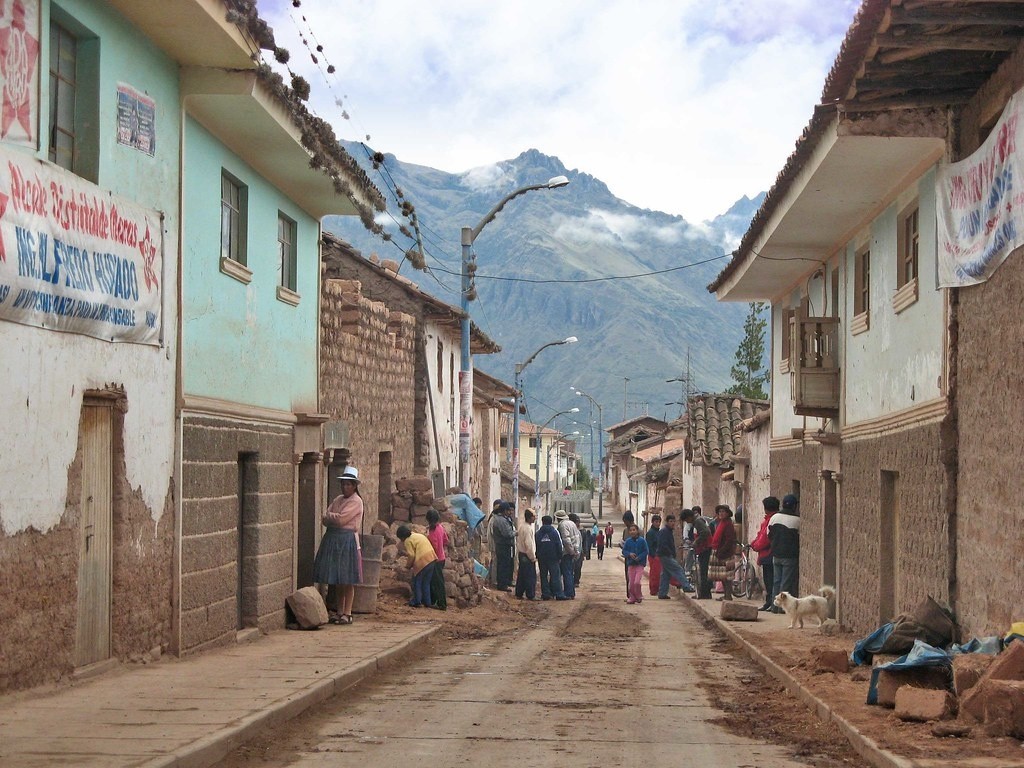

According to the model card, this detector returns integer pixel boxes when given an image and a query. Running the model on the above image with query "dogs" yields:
[774,586,836,629]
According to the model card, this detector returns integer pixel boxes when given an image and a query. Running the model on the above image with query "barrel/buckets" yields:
[326,533,384,614]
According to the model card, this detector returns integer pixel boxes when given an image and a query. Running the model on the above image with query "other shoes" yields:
[758,605,766,611]
[658,596,669,599]
[766,606,771,611]
[684,589,695,593]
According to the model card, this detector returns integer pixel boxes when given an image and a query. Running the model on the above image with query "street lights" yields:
[514,335,579,522]
[575,392,602,518]
[546,431,579,516]
[570,386,594,498]
[459,173,569,492]
[536,408,580,523]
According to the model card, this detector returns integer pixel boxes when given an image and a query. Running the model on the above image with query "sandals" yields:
[330,614,341,624]
[335,614,353,625]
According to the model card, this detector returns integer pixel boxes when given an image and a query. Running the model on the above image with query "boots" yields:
[715,586,732,601]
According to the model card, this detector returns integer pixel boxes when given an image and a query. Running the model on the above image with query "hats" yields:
[783,495,799,505]
[337,466,361,485]
[553,510,568,519]
[715,504,733,517]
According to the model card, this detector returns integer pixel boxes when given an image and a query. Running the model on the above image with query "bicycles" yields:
[678,545,701,599]
[731,540,755,599]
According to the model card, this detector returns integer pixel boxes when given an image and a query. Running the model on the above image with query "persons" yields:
[750,494,801,614]
[312,465,363,624]
[535,510,586,601]
[396,526,438,607]
[680,504,742,602]
[619,510,648,604]
[473,497,541,601]
[585,522,613,561]
[645,514,695,599]
[426,509,449,611]
